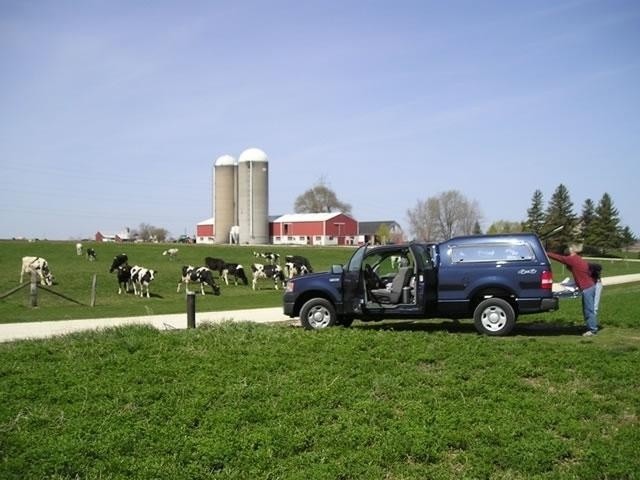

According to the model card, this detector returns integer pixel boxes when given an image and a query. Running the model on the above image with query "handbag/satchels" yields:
[589,263,601,277]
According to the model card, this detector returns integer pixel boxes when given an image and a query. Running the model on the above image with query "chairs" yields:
[371,258,413,303]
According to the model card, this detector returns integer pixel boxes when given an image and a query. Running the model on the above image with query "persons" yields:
[545,241,600,337]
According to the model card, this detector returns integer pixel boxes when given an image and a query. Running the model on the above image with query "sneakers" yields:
[583,331,594,337]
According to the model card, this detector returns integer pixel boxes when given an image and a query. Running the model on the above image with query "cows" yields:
[19,256,55,287]
[75,242,84,256]
[162,248,179,263]
[85,247,97,262]
[108,253,158,299]
[177,247,314,297]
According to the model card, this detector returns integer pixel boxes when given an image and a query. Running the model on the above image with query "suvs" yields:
[282,223,567,338]
